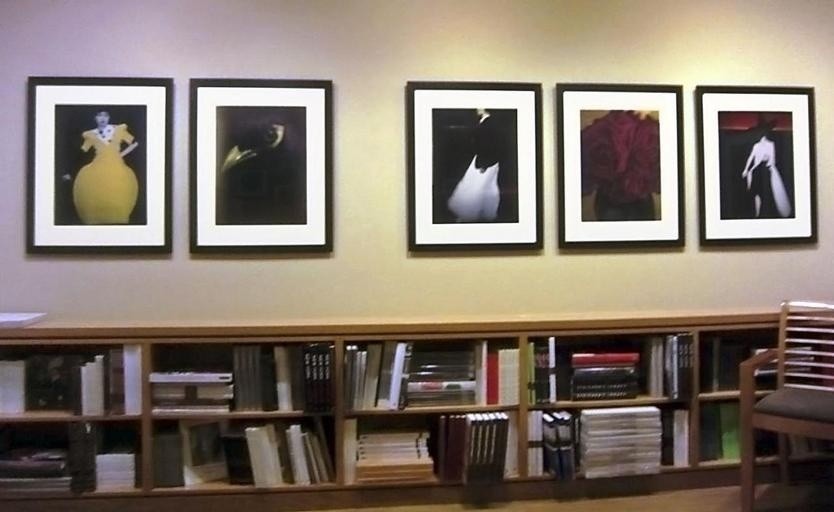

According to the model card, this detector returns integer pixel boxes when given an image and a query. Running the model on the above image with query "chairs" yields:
[735,301,833,512]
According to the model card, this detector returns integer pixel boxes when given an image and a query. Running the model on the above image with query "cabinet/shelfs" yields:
[1,301,834,512]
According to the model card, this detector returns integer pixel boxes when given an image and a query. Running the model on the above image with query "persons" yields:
[737,116,782,219]
[446,109,507,223]
[76,107,141,226]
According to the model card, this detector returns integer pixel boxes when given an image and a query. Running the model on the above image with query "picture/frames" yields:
[189,76,334,255]
[405,80,544,251]
[556,81,687,250]
[694,84,817,248]
[23,74,173,255]
